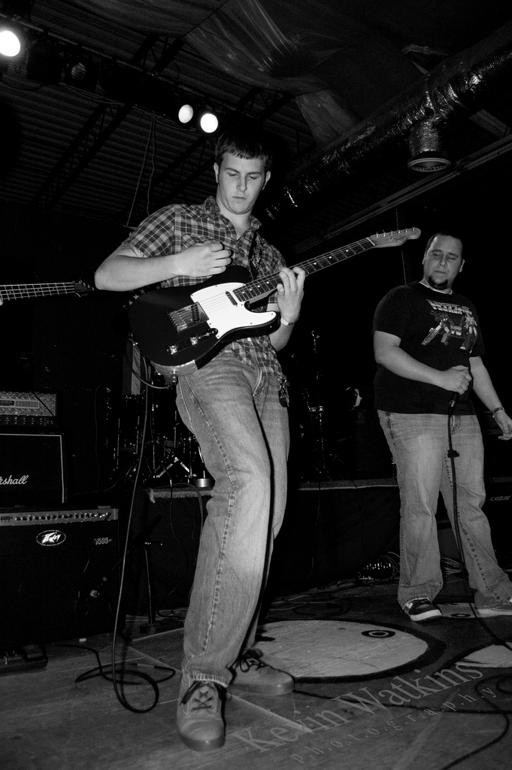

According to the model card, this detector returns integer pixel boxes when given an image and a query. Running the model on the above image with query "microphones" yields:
[449,368,469,408]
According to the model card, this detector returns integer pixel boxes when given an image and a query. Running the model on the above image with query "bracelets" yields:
[491,406,504,419]
[278,317,299,329]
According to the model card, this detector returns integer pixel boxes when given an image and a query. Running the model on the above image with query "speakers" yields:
[0,508,125,652]
[0,433,64,510]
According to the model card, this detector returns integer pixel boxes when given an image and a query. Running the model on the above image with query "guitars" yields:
[127,226,421,378]
[0,280,96,303]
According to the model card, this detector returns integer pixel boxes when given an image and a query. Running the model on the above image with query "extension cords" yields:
[0,642,48,673]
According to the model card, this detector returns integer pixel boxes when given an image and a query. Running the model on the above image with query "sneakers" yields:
[475,595,512,618]
[403,598,442,622]
[175,670,227,752]
[227,655,295,697]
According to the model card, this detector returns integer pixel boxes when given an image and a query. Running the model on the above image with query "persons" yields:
[89,123,308,757]
[370,227,512,629]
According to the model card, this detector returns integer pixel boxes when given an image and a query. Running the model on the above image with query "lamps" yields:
[407,117,451,175]
